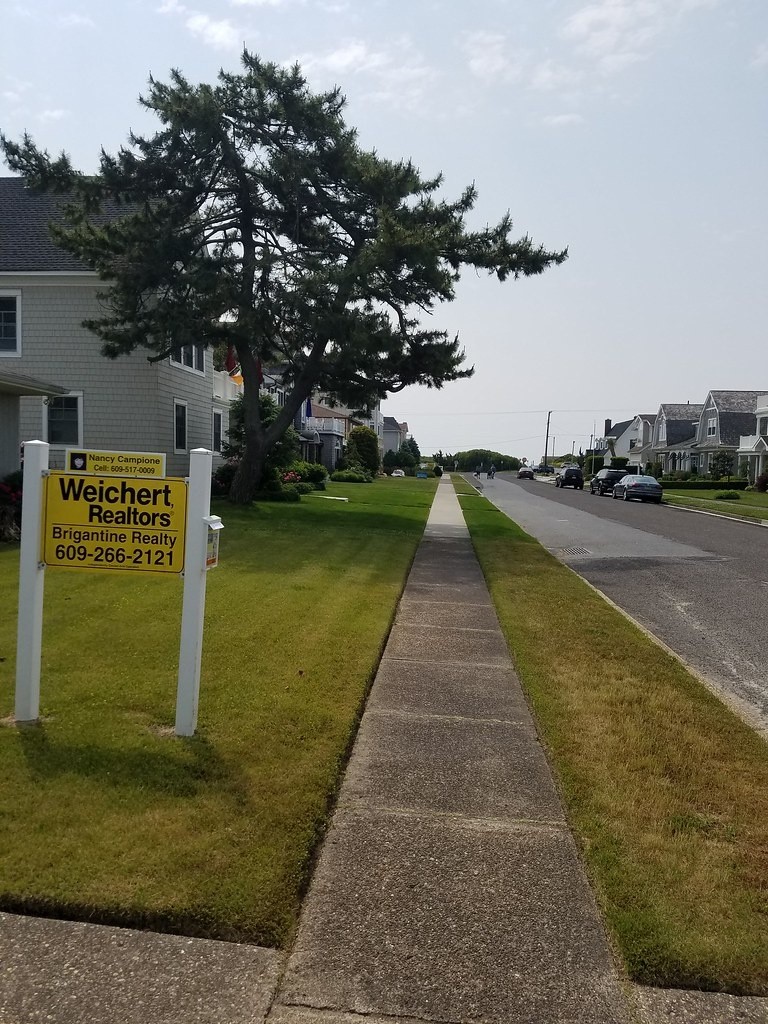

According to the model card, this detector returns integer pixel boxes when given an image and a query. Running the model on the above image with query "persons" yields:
[477,466,481,479]
[71,454,87,470]
[487,463,496,479]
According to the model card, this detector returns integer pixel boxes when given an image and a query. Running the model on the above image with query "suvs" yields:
[589,469,630,496]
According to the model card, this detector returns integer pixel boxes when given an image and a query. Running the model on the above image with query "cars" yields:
[517,467,534,480]
[532,466,554,474]
[560,462,577,469]
[556,468,584,490]
[612,475,663,504]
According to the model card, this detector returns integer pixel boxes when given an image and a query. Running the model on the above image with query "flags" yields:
[222,325,244,387]
